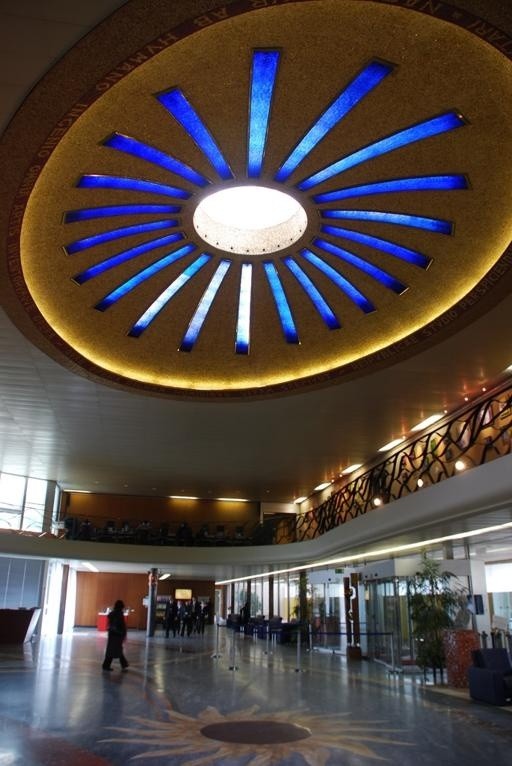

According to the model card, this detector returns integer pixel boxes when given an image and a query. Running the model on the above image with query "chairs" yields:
[63,517,275,547]
[464,648,512,706]
[227,613,283,639]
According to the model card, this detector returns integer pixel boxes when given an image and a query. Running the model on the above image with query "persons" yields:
[163,594,208,638]
[102,600,132,671]
